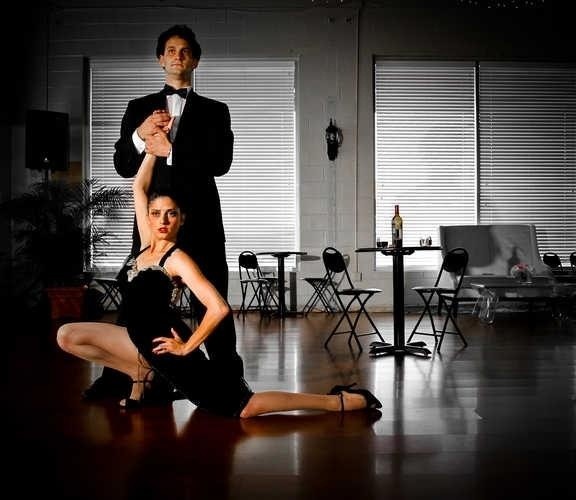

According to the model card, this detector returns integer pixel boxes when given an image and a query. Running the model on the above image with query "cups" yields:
[377,236,388,248]
[420,234,432,246]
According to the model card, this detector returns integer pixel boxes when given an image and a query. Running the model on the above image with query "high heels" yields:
[328,383,382,412]
[118,368,160,408]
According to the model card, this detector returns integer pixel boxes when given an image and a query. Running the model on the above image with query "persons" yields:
[55,107,384,421]
[77,23,253,402]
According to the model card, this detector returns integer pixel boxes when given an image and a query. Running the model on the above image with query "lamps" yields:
[325,119,343,160]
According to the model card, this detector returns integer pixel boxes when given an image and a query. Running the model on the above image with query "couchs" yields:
[437,225,555,318]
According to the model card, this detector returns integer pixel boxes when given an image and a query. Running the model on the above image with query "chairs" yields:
[355,246,443,357]
[407,247,469,352]
[322,246,386,353]
[543,252,576,274]
[92,255,194,319]
[236,251,335,322]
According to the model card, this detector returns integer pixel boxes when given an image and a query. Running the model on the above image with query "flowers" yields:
[511,264,536,278]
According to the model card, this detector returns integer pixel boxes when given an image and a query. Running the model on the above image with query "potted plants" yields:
[0,177,131,334]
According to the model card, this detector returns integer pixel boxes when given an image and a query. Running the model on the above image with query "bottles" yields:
[391,205,404,248]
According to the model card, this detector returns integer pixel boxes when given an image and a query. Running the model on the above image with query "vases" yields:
[520,269,533,283]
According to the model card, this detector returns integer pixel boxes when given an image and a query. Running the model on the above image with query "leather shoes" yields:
[237,376,252,392]
[80,376,131,398]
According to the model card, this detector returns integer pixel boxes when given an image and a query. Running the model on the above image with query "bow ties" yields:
[164,84,187,99]
